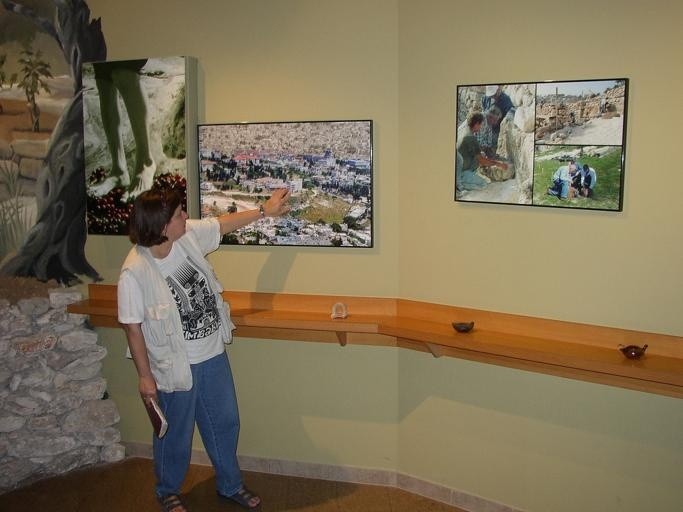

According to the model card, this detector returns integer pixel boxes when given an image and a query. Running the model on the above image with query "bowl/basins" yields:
[451,321,475,333]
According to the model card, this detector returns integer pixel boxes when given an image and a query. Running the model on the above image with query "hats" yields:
[485,85,499,96]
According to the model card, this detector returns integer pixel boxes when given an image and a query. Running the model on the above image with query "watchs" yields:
[258,204,265,218]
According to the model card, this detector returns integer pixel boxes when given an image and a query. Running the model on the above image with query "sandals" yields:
[217,486,261,508]
[157,493,186,512]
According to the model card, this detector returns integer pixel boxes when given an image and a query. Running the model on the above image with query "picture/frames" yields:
[195,118,374,250]
[451,77,630,211]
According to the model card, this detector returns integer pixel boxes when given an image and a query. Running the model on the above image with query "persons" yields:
[116,187,292,512]
[86,58,156,204]
[455,84,514,197]
[545,158,597,202]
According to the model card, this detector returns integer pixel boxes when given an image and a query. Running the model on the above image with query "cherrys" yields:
[87,166,186,234]
